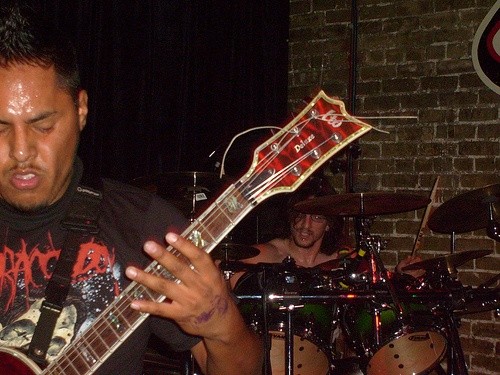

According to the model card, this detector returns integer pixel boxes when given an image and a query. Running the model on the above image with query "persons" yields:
[214,177,427,374]
[0,3,265,375]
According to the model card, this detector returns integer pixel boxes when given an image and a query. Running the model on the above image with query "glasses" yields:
[295,212,327,223]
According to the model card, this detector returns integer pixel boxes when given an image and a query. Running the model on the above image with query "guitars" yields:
[0,88,374,375]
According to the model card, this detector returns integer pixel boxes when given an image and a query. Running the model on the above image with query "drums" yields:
[338,273,432,363]
[246,321,337,375]
[366,329,460,375]
[233,265,333,345]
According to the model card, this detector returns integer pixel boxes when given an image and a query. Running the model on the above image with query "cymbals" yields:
[427,184,500,234]
[293,191,432,217]
[125,171,227,202]
[210,242,261,262]
[401,248,495,270]
